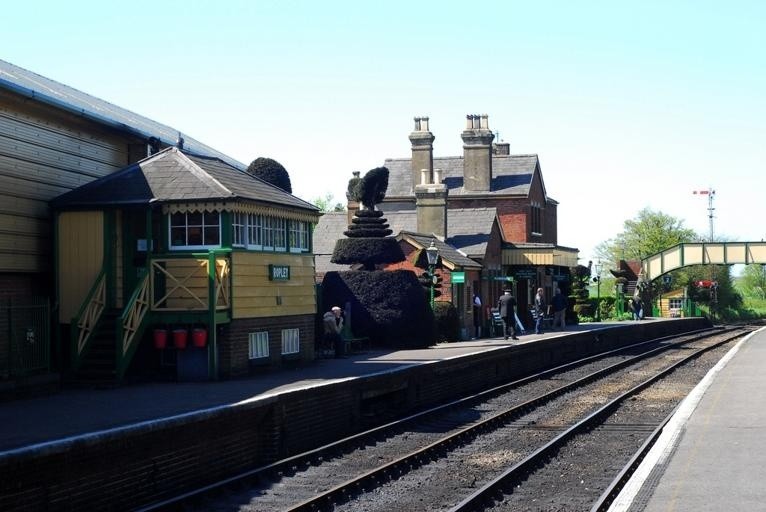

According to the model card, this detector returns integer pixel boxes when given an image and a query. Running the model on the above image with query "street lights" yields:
[425,239,439,346]
[592,261,603,323]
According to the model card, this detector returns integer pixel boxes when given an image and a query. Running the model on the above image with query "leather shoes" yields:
[504,337,519,340]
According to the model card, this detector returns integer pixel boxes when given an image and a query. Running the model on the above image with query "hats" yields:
[504,289,511,292]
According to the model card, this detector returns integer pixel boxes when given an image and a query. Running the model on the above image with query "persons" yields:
[535,288,545,334]
[497,287,520,340]
[551,287,569,331]
[632,296,643,321]
[473,293,482,337]
[321,305,348,359]
